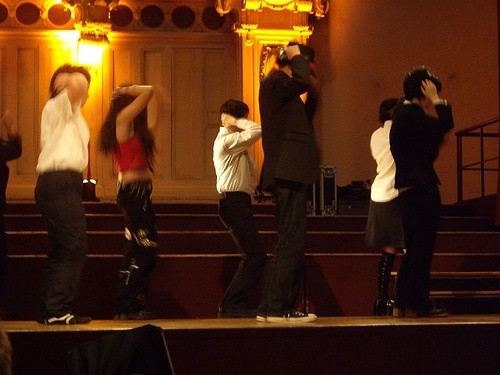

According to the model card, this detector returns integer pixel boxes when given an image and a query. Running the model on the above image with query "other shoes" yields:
[403,303,448,317]
[392,305,404,317]
[44,309,91,324]
[114,309,159,320]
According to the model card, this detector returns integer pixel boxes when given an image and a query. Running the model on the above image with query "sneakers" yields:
[256,307,267,322]
[266,310,318,323]
[218,305,257,318]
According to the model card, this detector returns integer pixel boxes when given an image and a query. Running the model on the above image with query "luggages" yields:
[313,167,337,216]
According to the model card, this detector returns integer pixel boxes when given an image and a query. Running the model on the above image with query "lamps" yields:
[62,0,116,49]
[214,0,330,82]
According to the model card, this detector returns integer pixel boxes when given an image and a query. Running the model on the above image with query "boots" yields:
[373,249,395,315]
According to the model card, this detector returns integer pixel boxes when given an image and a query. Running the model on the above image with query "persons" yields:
[34,63,93,324]
[364,99,400,316]
[390,66,455,319]
[212,100,267,318]
[98,82,159,320]
[258,42,321,322]
